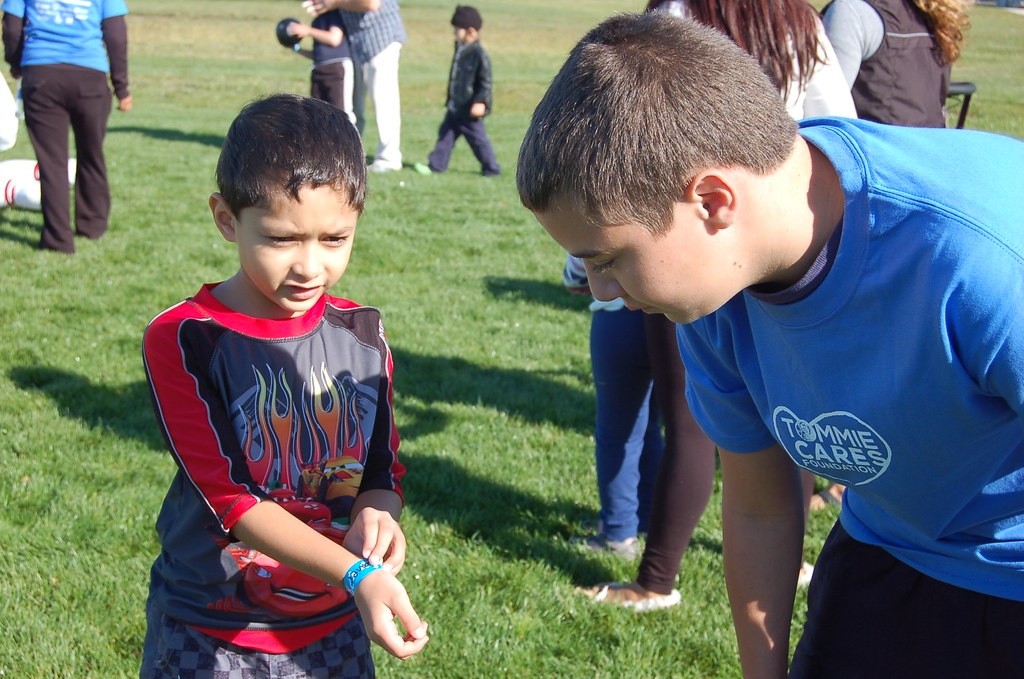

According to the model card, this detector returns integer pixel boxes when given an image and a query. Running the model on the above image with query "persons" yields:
[574,1,860,611]
[0,0,133,254]
[513,10,1024,679]
[821,0,969,128]
[140,94,429,678]
[286,0,405,175]
[415,5,500,178]
[554,250,669,562]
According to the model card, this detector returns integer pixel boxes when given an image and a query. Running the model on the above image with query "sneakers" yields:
[562,530,640,565]
[367,162,405,173]
[797,559,816,589]
[414,164,434,176]
[575,581,683,615]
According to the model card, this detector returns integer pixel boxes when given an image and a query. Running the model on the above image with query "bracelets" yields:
[343,557,381,595]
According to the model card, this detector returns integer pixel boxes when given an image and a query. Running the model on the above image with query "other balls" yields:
[277,19,301,47]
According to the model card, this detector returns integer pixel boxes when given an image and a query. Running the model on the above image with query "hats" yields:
[450,6,483,31]
